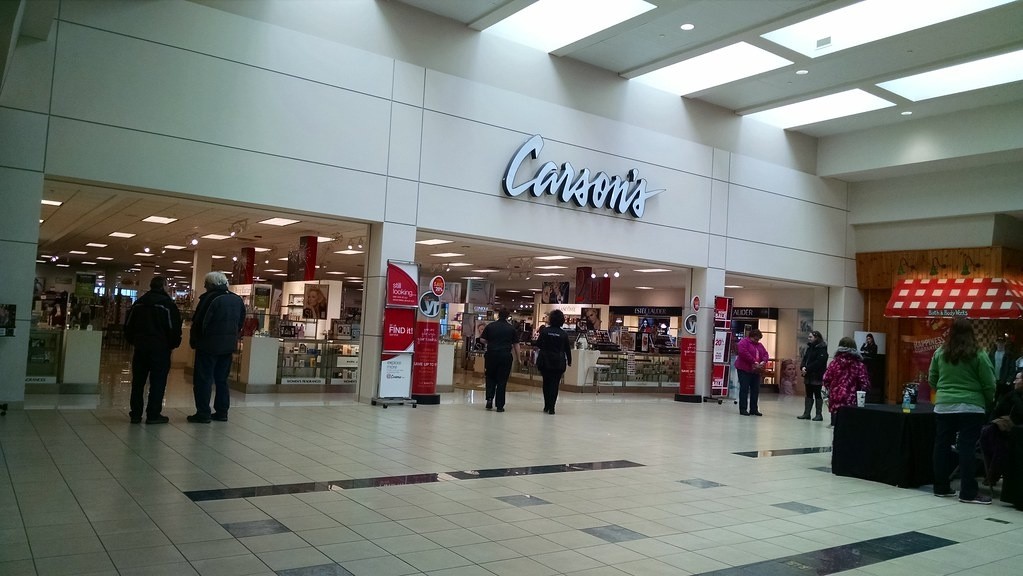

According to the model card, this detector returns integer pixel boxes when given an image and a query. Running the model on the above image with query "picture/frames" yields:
[302,283,329,320]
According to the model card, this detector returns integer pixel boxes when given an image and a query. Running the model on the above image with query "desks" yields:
[831,404,951,489]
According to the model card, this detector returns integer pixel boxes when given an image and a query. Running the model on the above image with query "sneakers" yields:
[959,492,992,504]
[934,488,956,497]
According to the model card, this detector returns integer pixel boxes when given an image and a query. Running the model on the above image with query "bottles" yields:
[902,391,911,414]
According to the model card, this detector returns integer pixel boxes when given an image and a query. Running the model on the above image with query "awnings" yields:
[883,278,1023,321]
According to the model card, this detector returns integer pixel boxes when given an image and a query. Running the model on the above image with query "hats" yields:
[996,336,1006,342]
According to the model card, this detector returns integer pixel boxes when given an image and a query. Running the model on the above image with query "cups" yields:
[857,390,866,407]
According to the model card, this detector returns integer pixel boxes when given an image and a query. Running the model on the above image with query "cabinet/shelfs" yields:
[228,337,360,393]
[511,344,681,393]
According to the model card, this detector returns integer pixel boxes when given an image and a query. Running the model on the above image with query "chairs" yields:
[762,358,775,384]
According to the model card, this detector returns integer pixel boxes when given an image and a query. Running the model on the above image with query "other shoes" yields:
[130,417,142,423]
[211,411,228,421]
[146,414,169,424]
[544,407,555,414]
[187,412,211,423]
[486,398,492,409]
[750,410,762,416]
[497,407,505,412]
[740,411,750,415]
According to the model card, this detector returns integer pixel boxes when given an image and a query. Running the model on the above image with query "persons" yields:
[532,310,573,418]
[479,308,524,414]
[638,318,652,334]
[477,324,486,336]
[950,333,1023,511]
[859,333,878,397]
[796,330,829,422]
[822,337,872,427]
[302,288,327,320]
[123,275,182,426]
[542,282,565,304]
[779,359,797,396]
[923,318,998,506]
[584,309,601,329]
[732,329,769,417]
[185,272,247,424]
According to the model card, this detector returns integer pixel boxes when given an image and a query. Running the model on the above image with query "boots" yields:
[812,398,823,421]
[796,397,814,419]
